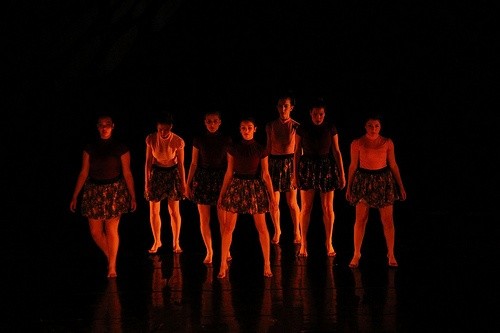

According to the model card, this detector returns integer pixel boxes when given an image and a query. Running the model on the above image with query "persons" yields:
[265,95,303,246]
[145,116,187,253]
[70,114,137,279]
[345,117,407,269]
[293,103,346,257]
[185,107,234,264]
[217,115,279,279]
